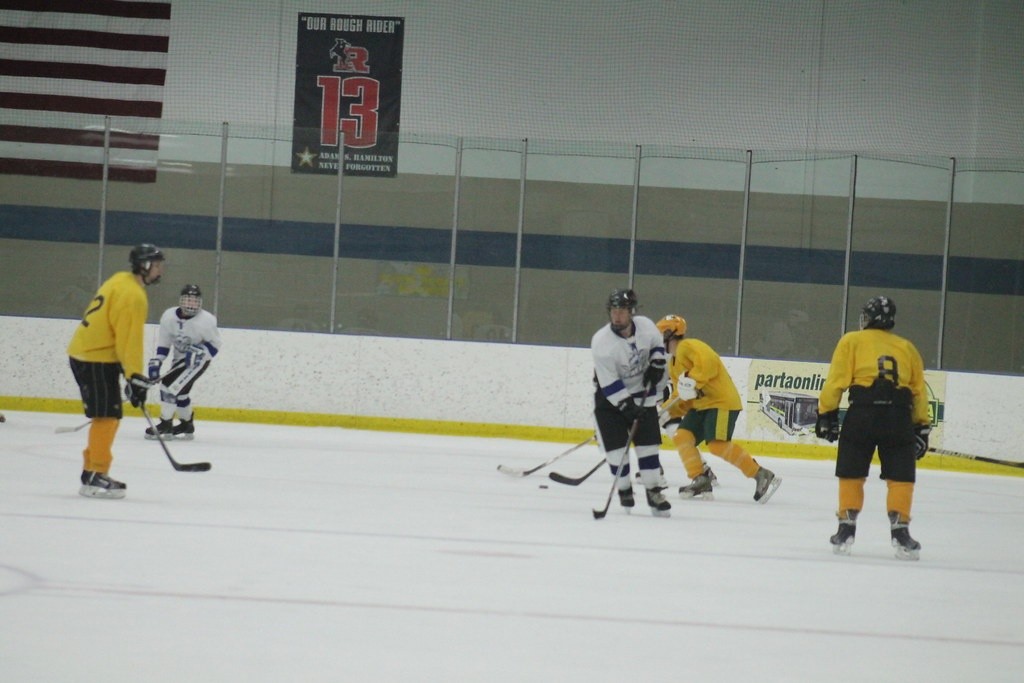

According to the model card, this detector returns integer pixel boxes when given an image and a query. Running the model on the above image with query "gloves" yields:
[656,405,671,427]
[148,358,162,385]
[124,373,149,408]
[914,423,932,460]
[185,344,203,368]
[616,396,647,426]
[676,370,698,401]
[814,407,841,443]
[642,359,666,389]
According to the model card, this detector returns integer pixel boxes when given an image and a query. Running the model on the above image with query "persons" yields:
[67,244,165,493]
[591,287,717,513]
[656,314,781,502]
[815,296,932,553]
[145,284,222,436]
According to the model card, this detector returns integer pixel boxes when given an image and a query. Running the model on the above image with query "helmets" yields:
[128,244,165,271]
[606,289,638,308]
[859,296,896,331]
[179,283,203,317]
[655,314,686,338]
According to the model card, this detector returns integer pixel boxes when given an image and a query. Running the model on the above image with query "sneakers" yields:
[752,458,782,504]
[678,462,718,502]
[78,468,127,499]
[888,510,921,560]
[830,508,859,555]
[618,482,635,515]
[646,486,672,517]
[145,410,195,441]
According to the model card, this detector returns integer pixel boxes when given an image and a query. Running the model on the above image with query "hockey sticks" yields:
[820,425,1024,469]
[549,395,679,486]
[124,378,212,473]
[56,361,186,434]
[496,434,596,479]
[592,379,653,519]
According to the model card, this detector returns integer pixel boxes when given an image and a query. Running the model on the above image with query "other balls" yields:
[539,485,548,488]
